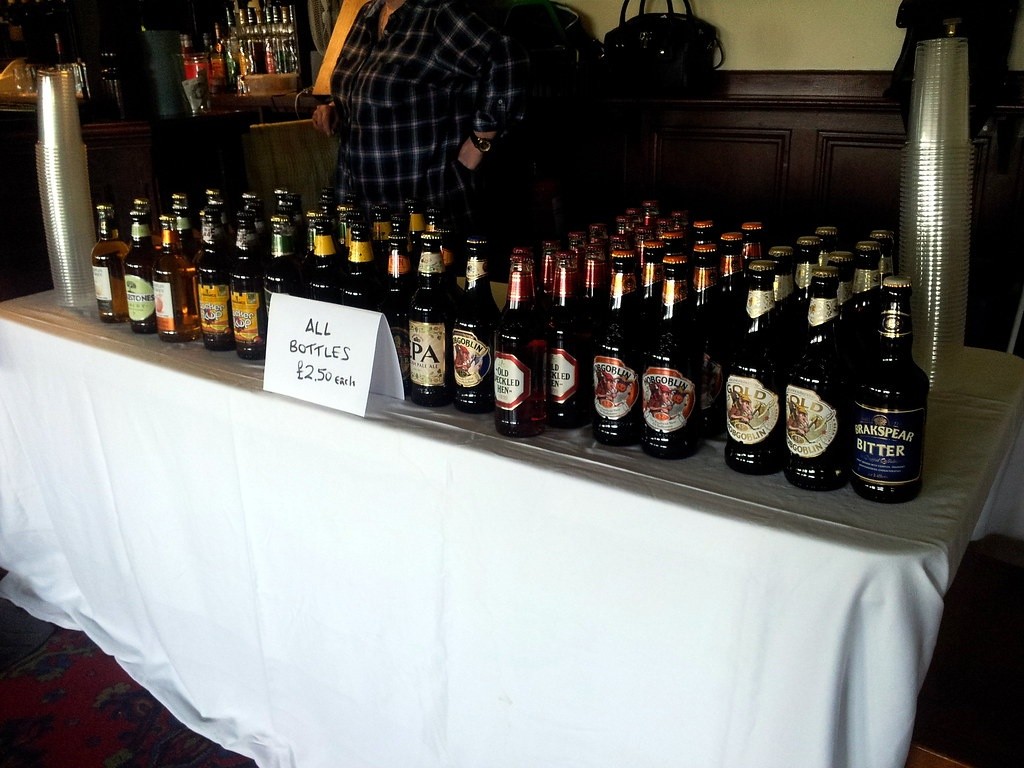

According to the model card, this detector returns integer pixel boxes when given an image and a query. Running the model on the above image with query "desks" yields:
[0,286,1023,768]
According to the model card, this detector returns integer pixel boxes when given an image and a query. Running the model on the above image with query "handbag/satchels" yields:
[0,57,91,108]
[604,0,717,99]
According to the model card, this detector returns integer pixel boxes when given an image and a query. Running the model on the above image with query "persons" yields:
[312,0,517,240]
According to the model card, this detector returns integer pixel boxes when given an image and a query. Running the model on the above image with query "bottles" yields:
[189,1,296,98]
[88,179,935,508]
[36,25,135,123]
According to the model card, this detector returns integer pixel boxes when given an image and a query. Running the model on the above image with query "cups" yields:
[892,34,976,384]
[34,67,102,310]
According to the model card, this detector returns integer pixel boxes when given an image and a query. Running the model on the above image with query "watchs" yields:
[469,131,491,152]
